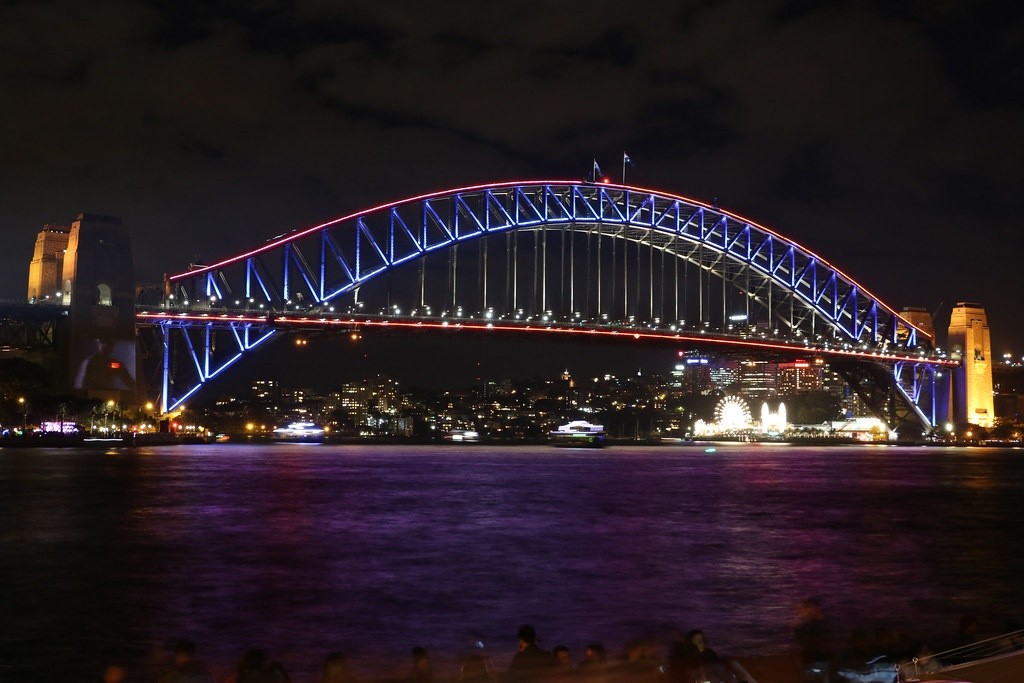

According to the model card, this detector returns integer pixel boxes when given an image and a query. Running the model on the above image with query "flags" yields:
[624,154,630,162]
[594,161,599,170]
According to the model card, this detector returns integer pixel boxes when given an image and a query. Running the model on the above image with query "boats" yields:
[548,419,611,448]
[274,422,324,443]
